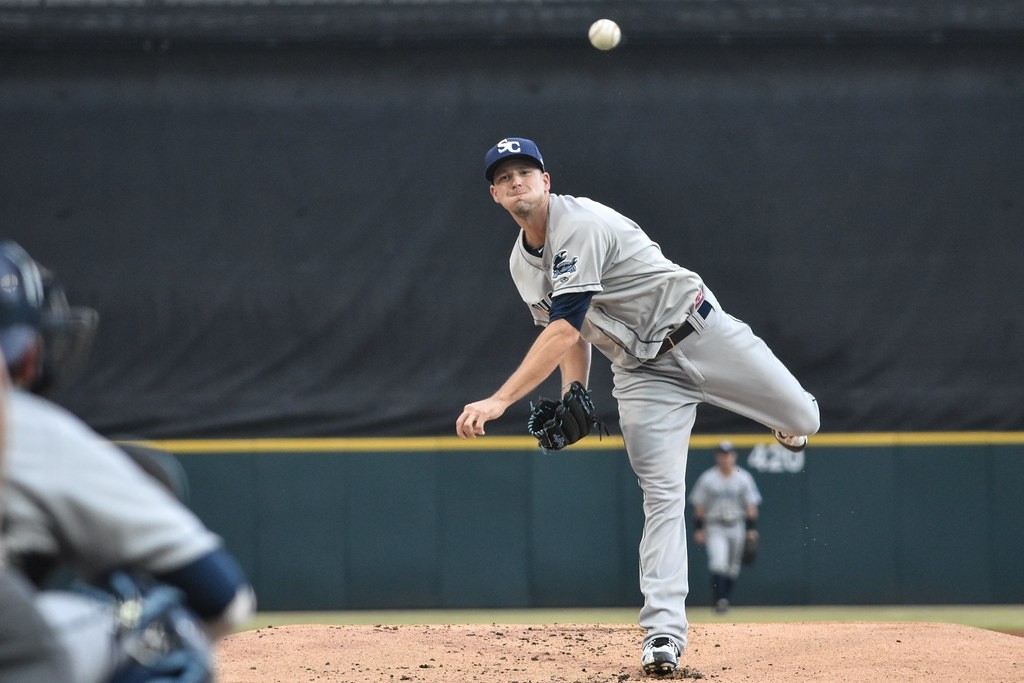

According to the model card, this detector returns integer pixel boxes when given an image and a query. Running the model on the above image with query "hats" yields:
[716,440,736,452]
[484,138,544,181]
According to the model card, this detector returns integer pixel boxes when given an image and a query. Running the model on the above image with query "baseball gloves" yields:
[527,378,597,455]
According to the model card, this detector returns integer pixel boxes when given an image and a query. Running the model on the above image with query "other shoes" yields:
[712,596,728,615]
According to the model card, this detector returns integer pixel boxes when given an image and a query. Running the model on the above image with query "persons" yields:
[0,238,255,683]
[456,138,821,677]
[688,443,762,613]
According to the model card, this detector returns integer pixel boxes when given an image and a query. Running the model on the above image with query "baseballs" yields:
[588,17,624,51]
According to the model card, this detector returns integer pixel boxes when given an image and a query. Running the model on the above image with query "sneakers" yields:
[640,637,680,674]
[773,430,807,452]
